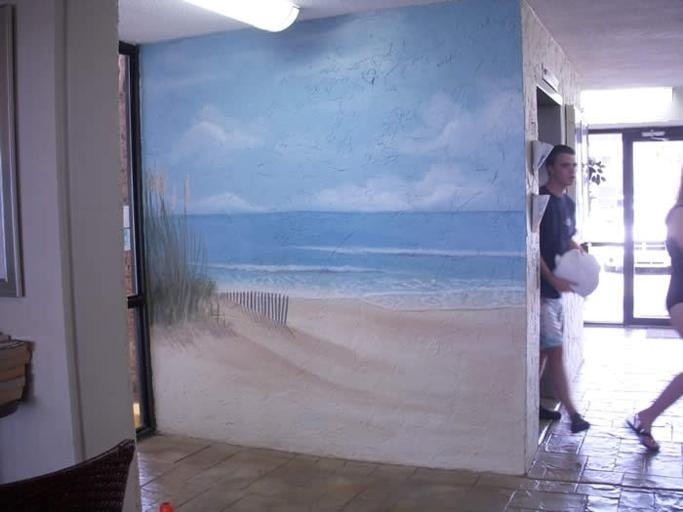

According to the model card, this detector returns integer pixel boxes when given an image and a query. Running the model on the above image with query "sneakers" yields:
[567,414,590,433]
[540,406,562,421]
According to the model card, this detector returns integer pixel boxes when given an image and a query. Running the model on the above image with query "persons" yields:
[540,145,591,434]
[624,164,683,454]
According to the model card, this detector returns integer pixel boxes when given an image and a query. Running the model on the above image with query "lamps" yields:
[176,0,300,35]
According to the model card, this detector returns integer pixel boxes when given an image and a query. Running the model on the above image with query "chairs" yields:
[0,436,136,512]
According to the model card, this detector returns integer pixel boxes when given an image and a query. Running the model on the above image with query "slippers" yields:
[625,413,660,452]
